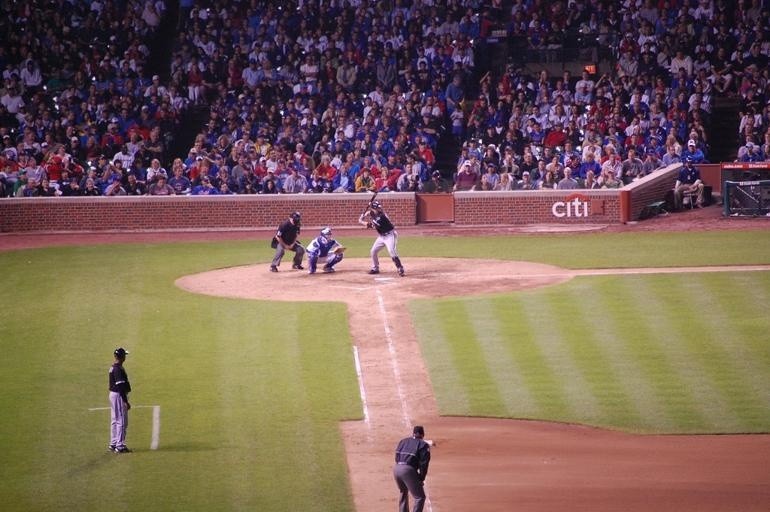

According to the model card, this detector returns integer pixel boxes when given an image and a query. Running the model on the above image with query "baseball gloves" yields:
[334,246,346,254]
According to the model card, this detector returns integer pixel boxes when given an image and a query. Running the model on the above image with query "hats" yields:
[413,426,424,434]
[368,201,381,208]
[113,347,129,356]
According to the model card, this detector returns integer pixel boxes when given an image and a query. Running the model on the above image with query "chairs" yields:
[671,177,700,210]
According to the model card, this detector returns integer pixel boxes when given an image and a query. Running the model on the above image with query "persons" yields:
[391,425,430,512]
[108,348,132,453]
[0,0,769,196]
[673,156,704,210]
[306,226,343,273]
[270,212,305,273]
[359,201,405,275]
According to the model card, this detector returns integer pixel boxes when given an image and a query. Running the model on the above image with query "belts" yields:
[396,460,417,470]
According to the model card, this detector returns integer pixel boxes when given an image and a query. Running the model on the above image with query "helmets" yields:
[320,228,332,243]
[288,212,302,226]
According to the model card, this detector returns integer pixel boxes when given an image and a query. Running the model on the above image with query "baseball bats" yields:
[364,192,377,214]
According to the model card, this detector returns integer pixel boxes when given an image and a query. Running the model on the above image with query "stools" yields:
[646,200,667,219]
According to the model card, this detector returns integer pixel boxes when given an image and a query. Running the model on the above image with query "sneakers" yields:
[270,264,406,277]
[109,444,131,454]
